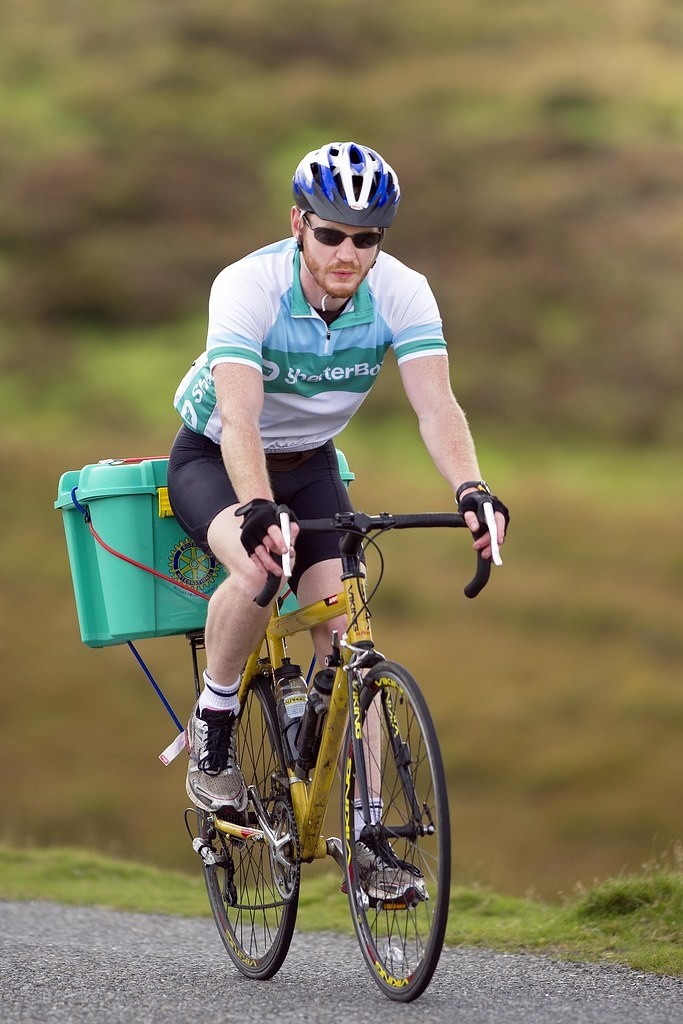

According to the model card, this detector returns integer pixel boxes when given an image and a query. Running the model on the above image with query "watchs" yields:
[454,480,491,505]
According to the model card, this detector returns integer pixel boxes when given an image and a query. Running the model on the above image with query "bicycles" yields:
[184,503,504,1004]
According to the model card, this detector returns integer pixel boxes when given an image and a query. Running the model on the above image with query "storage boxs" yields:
[52,447,355,651]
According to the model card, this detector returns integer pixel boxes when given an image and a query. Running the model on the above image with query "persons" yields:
[168,141,510,905]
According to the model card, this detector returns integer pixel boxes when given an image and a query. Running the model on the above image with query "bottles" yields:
[294,669,335,759]
[274,656,310,771]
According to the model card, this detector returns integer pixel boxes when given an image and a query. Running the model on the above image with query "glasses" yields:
[298,208,384,249]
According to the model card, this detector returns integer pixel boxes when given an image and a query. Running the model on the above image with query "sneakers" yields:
[341,840,429,904]
[186,699,248,813]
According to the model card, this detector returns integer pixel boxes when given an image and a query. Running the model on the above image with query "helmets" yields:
[292,141,401,228]
